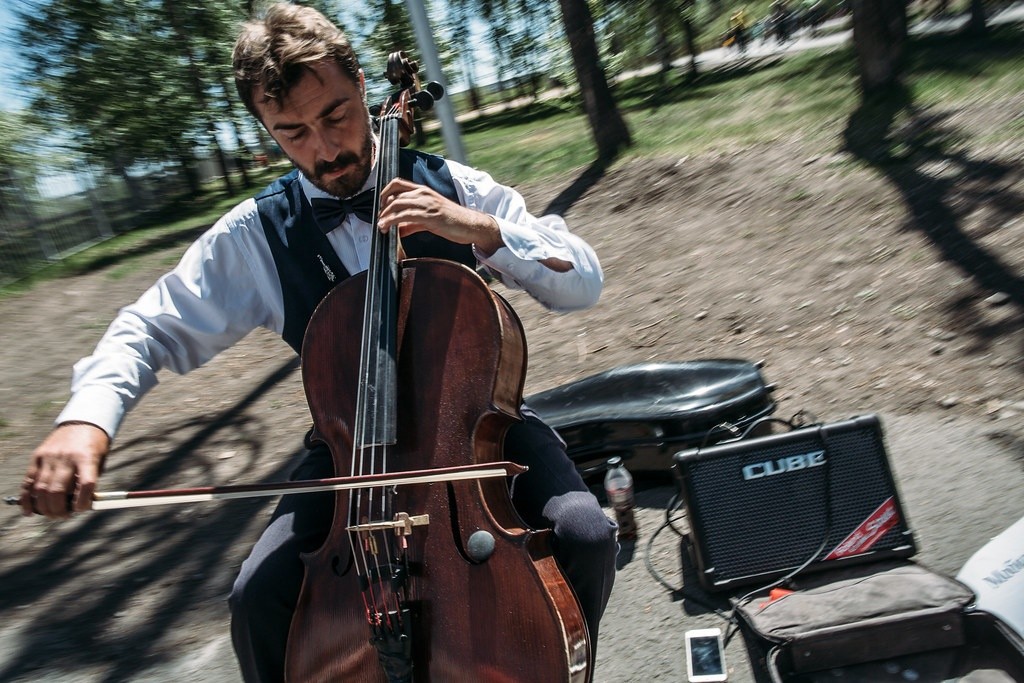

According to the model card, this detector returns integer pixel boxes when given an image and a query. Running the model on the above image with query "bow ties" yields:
[311,187,375,234]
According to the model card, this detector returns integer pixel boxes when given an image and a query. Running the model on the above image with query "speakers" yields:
[673,415,919,590]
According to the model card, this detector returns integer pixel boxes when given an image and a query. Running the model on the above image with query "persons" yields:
[21,0,621,683]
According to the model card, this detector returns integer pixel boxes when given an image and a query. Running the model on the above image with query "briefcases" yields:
[725,556,1024,682]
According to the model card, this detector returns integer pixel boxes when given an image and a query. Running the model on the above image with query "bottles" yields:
[604,456,637,541]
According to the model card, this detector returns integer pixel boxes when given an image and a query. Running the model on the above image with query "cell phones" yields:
[684,627,727,681]
